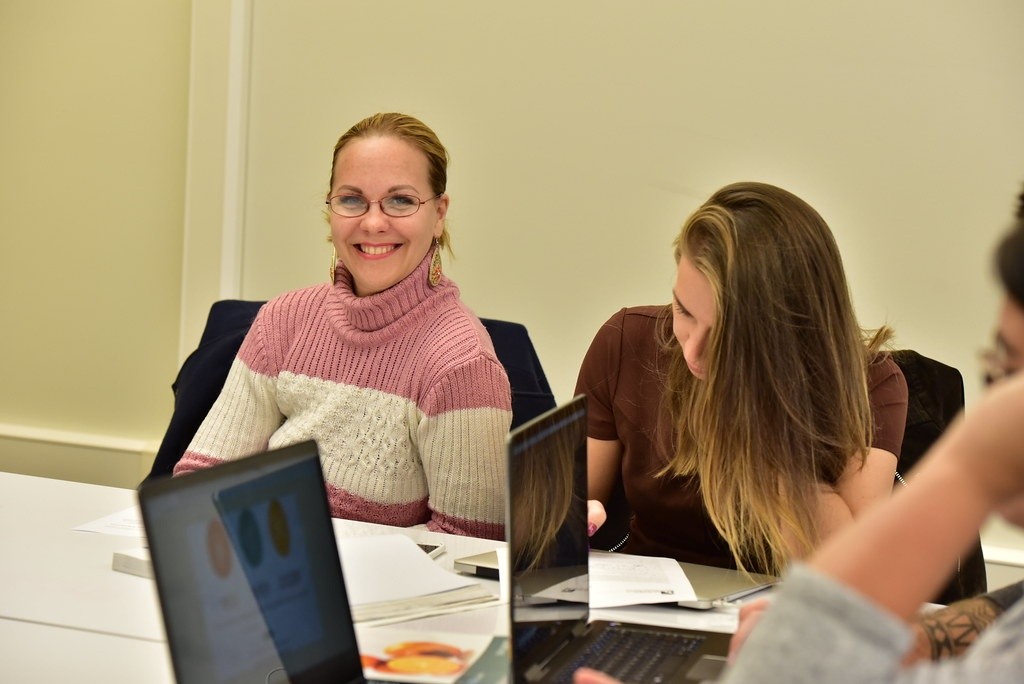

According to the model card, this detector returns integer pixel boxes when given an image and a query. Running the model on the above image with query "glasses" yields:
[325,192,442,219]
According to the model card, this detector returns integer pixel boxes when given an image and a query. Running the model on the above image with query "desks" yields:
[0,472,946,684]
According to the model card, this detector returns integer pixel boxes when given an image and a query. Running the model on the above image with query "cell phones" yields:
[413,538,444,559]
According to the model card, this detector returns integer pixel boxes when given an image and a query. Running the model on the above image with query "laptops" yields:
[504,393,735,683]
[134,440,446,684]
[452,544,776,609]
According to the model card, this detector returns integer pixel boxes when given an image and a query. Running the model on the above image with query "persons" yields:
[170,112,514,540]
[573,182,1022,684]
